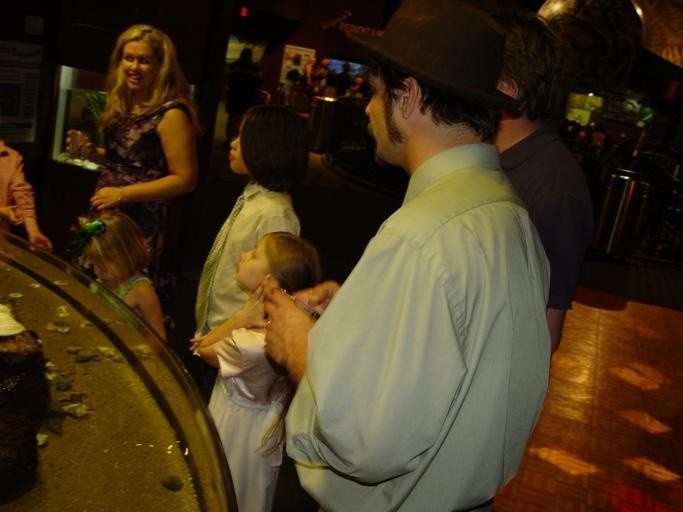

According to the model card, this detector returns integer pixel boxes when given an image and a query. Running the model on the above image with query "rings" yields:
[264,320,271,329]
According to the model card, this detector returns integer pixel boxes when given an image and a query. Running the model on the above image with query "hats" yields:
[344,1,521,109]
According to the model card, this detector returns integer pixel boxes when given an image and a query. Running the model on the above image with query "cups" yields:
[68,130,89,161]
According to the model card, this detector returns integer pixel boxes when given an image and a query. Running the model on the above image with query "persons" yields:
[224,47,264,139]
[190,228,325,511]
[262,0,558,512]
[66,23,200,260]
[181,101,316,398]
[76,207,167,344]
[0,140,54,256]
[286,47,369,98]
[491,8,596,353]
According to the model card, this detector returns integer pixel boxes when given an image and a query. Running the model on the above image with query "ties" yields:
[196,195,244,332]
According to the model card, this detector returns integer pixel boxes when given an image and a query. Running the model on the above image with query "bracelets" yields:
[118,185,126,202]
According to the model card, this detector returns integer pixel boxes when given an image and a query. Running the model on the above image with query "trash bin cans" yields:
[592,165,649,260]
